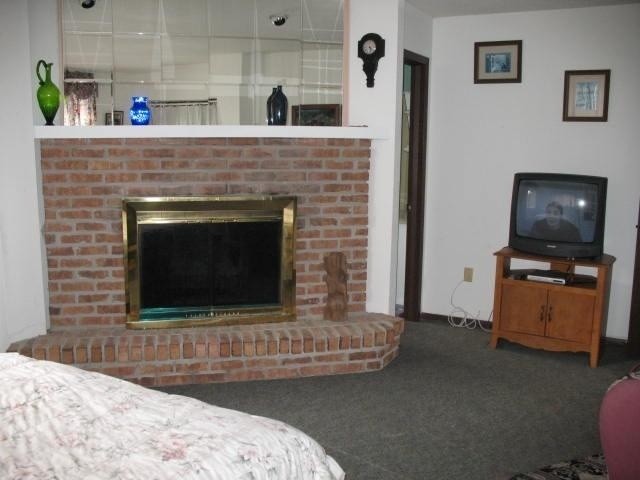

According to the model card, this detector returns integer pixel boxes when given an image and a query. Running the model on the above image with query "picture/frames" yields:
[473,39,523,85]
[562,68,610,123]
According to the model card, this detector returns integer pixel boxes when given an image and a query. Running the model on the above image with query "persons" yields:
[582,85,592,110]
[528,201,583,242]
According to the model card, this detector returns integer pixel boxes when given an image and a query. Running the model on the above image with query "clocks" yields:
[358,33,386,88]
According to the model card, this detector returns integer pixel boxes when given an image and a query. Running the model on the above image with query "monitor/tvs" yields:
[508,172,609,258]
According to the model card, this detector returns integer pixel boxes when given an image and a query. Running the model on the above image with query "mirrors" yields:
[55,1,352,127]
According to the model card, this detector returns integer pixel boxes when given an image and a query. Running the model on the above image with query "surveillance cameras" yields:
[79,0,97,9]
[269,13,290,27]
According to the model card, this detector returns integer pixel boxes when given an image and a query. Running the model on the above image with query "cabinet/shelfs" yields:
[488,246,616,370]
